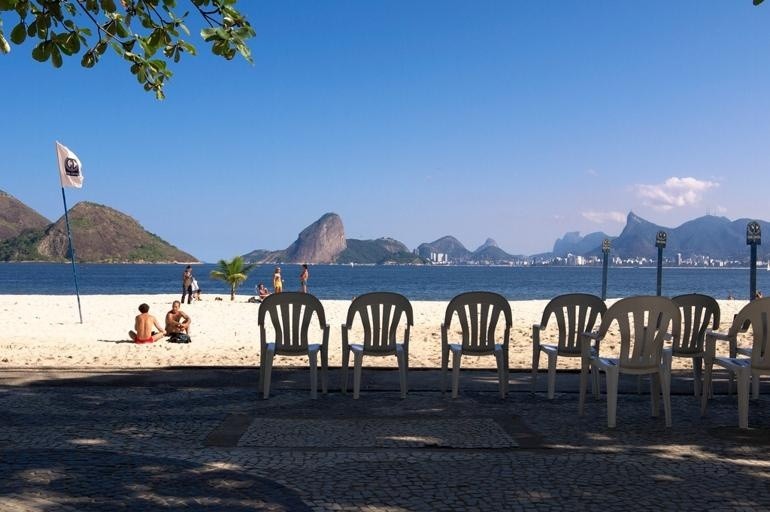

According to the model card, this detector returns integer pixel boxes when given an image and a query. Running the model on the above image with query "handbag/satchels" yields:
[168,332,191,343]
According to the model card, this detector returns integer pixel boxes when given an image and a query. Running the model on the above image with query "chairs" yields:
[578,296,680,429]
[532,293,604,400]
[342,292,414,400]
[700,296,770,432]
[640,294,714,400]
[441,291,512,399]
[257,292,331,400]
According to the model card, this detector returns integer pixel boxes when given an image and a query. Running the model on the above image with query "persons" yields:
[274,267,284,294]
[128,303,167,343]
[300,264,308,293]
[756,291,762,300]
[181,265,193,304]
[258,284,273,300]
[192,289,202,302]
[165,300,192,336]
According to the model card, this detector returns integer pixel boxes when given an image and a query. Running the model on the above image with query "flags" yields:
[55,142,83,189]
